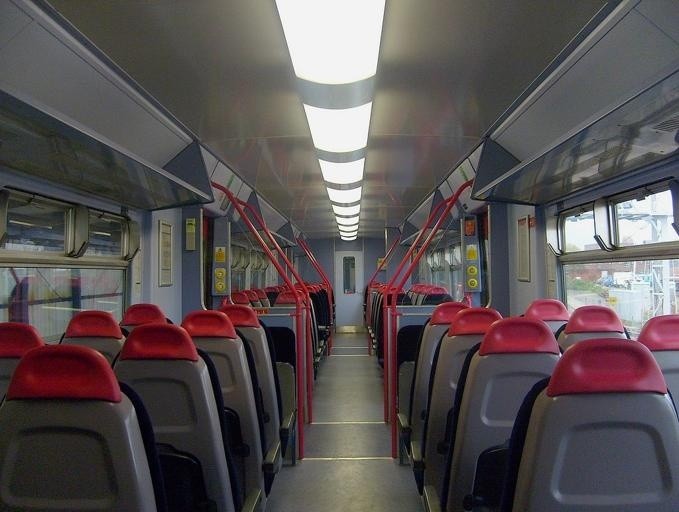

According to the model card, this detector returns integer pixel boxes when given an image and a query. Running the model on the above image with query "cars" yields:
[595,270,679,293]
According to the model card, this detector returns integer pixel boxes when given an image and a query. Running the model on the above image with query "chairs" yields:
[1,277,337,512]
[360,274,679,512]
